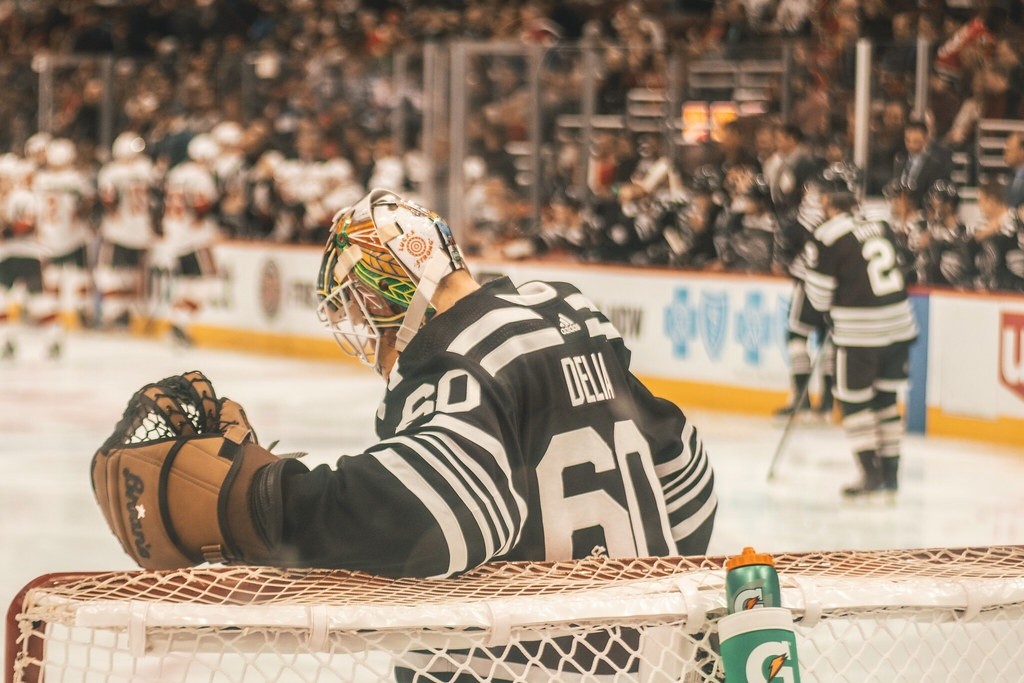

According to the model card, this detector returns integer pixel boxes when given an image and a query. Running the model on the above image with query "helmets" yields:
[313,186,472,381]
[21,118,242,165]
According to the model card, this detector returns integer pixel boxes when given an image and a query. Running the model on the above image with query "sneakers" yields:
[771,373,834,428]
[839,450,899,508]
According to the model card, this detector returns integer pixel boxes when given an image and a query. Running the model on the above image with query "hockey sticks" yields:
[765,330,833,484]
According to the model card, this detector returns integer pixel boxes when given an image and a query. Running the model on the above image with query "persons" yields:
[2,2,1024,367]
[90,188,719,681]
[790,190,920,498]
[771,166,839,426]
